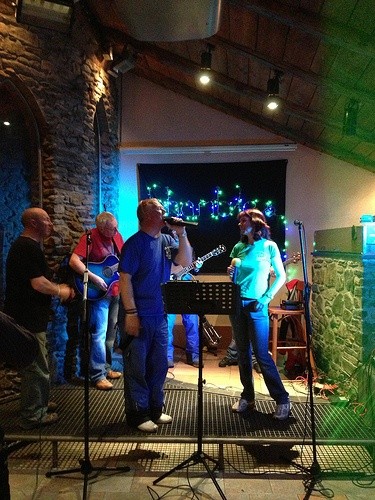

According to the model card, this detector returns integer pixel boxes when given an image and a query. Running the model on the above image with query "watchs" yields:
[265,292,274,300]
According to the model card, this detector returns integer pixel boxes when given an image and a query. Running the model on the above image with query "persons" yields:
[5,208,72,428]
[219,208,293,421]
[117,198,193,433]
[167,227,205,368]
[68,212,125,390]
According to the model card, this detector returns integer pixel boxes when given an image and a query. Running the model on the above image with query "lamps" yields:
[197,41,217,87]
[102,43,114,61]
[266,66,283,112]
[342,98,360,137]
[106,41,141,79]
[11,0,75,35]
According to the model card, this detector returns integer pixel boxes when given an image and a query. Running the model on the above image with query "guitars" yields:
[74,254,121,300]
[169,244,228,280]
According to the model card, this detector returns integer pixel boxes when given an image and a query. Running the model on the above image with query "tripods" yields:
[278,224,368,500]
[45,229,130,500]
[152,281,244,500]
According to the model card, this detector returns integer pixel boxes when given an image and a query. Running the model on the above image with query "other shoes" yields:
[94,378,114,390]
[18,402,61,430]
[272,401,294,421]
[135,419,159,433]
[155,413,173,424]
[106,370,122,379]
[254,363,262,373]
[188,363,204,368]
[231,398,256,413]
[218,357,239,367]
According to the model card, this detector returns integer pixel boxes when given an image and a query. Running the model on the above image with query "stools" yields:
[267,305,318,381]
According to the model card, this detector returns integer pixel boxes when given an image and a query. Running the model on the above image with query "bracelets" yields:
[124,308,138,316]
[177,232,187,237]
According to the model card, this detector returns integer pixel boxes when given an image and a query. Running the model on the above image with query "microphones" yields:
[294,219,302,226]
[167,218,199,226]
